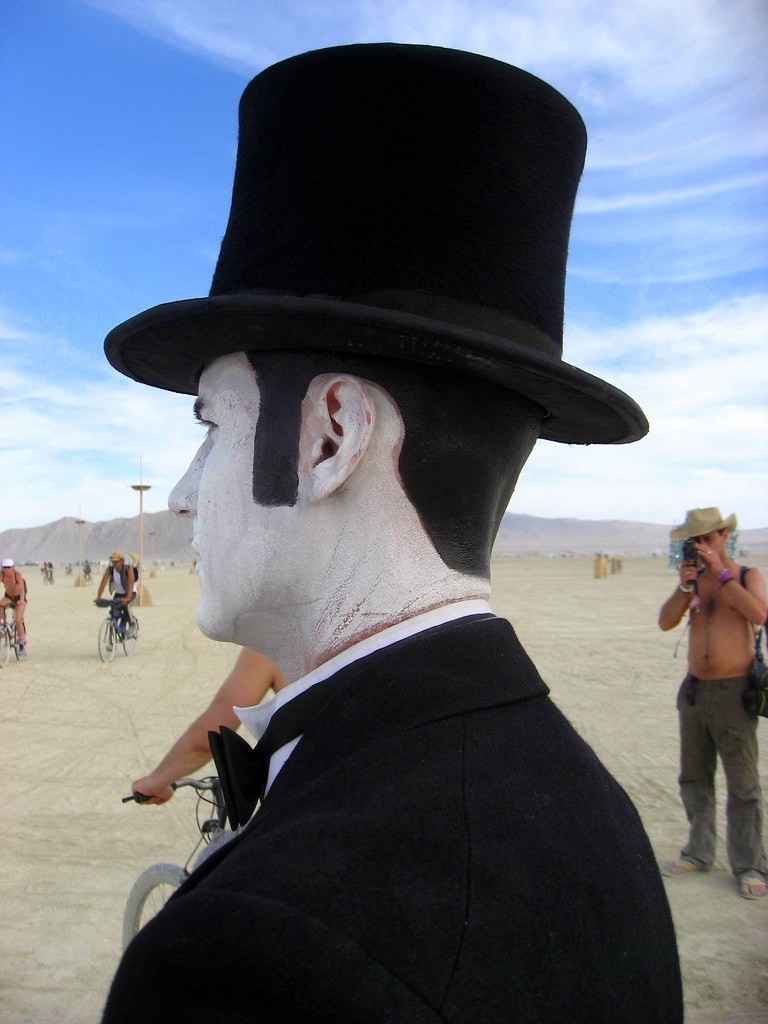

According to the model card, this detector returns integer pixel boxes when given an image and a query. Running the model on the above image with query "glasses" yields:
[1,566,11,569]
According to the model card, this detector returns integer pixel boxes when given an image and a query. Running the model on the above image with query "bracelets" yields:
[718,568,735,586]
[679,585,693,593]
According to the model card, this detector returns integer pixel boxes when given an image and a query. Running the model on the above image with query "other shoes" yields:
[127,621,136,637]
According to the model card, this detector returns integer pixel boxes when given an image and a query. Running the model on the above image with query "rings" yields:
[708,551,712,554]
[686,570,691,575]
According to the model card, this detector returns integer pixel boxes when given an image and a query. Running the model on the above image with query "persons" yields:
[83,560,91,579]
[132,647,289,805]
[658,507,768,901]
[0,559,26,655]
[96,551,137,651]
[103,44,683,1024]
[44,562,55,581]
[593,553,622,578]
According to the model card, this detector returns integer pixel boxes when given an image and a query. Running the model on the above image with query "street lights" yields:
[130,456,151,607]
[73,507,87,587]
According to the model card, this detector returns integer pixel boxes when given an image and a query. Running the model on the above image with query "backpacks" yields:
[109,565,139,589]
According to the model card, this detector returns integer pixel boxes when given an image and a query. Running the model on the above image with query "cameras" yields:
[684,539,699,586]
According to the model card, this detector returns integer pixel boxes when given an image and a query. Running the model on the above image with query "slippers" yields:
[659,858,697,877]
[738,876,768,900]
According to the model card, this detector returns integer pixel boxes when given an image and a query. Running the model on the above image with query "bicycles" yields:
[0,602,27,668]
[41,570,54,585]
[93,599,139,663]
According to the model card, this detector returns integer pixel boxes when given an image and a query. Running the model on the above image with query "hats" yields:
[669,507,738,538]
[109,551,125,562]
[2,558,14,566]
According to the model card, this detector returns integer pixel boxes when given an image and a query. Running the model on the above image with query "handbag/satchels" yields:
[739,565,768,717]
